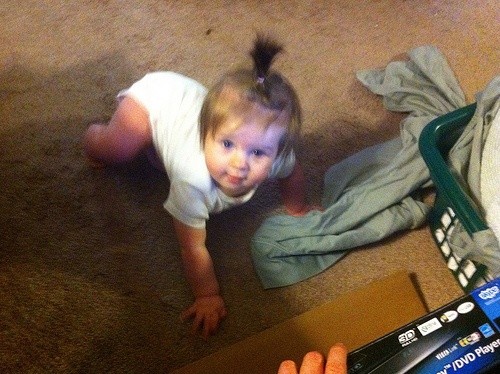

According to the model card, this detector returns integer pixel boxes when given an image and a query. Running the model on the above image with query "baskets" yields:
[418,102,489,294]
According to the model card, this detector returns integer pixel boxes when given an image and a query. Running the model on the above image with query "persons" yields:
[83,31,327,341]
